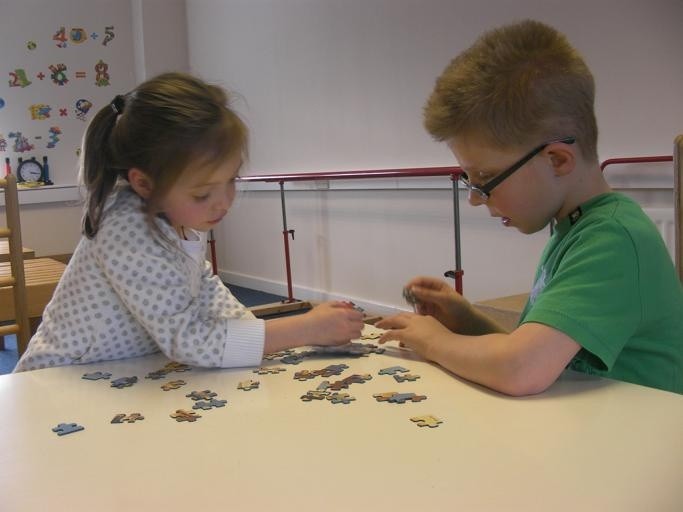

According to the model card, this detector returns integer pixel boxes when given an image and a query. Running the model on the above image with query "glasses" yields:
[460,136,575,200]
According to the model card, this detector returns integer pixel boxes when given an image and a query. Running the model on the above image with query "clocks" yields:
[16,160,42,182]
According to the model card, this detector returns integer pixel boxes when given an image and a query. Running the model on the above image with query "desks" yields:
[0,320,682,512]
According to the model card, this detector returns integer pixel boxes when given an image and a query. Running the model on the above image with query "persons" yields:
[374,17,682,396]
[11,71,365,374]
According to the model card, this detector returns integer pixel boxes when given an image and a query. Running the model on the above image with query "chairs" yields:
[0,175,30,359]
